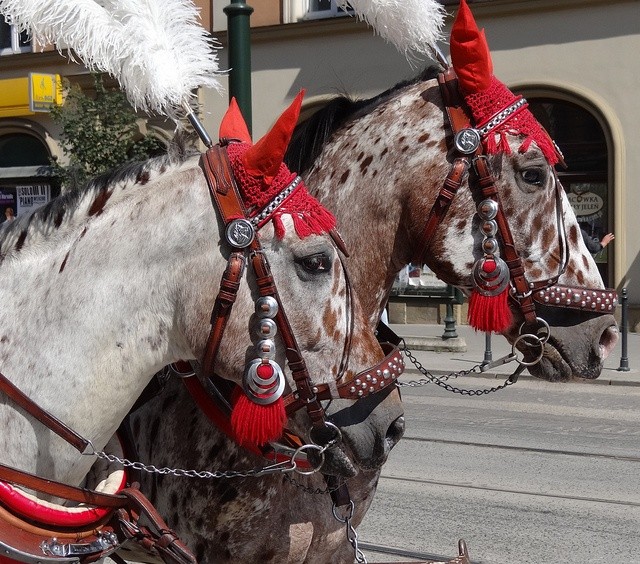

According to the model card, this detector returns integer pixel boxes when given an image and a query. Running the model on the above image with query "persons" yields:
[580,229,615,253]
[5,208,17,220]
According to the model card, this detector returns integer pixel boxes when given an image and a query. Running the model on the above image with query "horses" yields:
[0,91,405,563]
[96,0,619,563]
